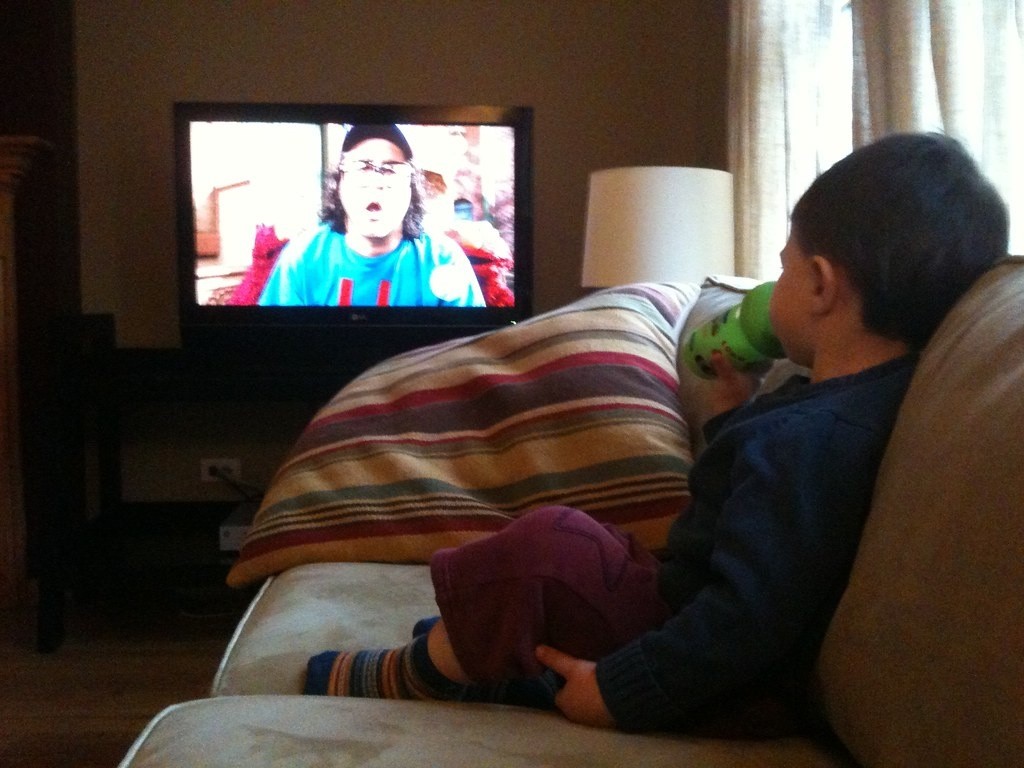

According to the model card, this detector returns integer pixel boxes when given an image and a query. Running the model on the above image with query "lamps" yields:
[581,165,742,288]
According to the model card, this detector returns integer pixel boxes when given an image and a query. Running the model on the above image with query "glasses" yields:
[338,160,415,183]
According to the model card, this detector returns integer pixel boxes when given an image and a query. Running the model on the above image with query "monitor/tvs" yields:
[172,99,534,350]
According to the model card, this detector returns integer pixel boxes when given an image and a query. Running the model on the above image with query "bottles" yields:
[684,281,788,379]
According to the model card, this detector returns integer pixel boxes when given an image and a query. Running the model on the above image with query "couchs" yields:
[118,249,1024,768]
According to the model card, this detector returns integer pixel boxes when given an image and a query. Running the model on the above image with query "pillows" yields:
[227,284,696,591]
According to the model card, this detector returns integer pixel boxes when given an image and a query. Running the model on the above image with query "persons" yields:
[304,127,1009,732]
[255,122,487,309]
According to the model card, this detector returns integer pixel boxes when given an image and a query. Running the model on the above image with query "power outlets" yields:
[200,457,243,484]
